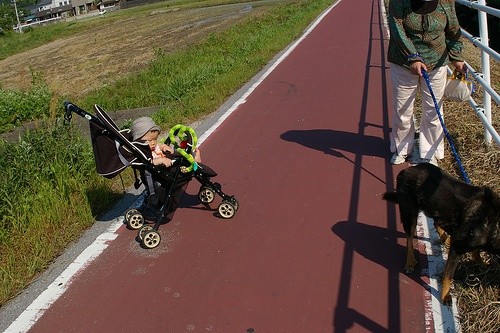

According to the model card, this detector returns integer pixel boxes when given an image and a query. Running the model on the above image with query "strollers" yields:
[64,102,238,249]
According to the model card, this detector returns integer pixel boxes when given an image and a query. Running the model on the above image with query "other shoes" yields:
[423,157,438,167]
[391,152,407,164]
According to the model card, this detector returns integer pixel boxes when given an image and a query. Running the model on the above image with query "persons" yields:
[387,0,467,165]
[131,117,202,168]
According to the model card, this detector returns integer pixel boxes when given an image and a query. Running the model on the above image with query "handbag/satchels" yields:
[443,67,473,102]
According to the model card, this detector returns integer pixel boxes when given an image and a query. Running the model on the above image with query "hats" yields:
[132,117,161,141]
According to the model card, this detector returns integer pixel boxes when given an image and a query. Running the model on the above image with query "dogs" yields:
[380,162,500,307]
